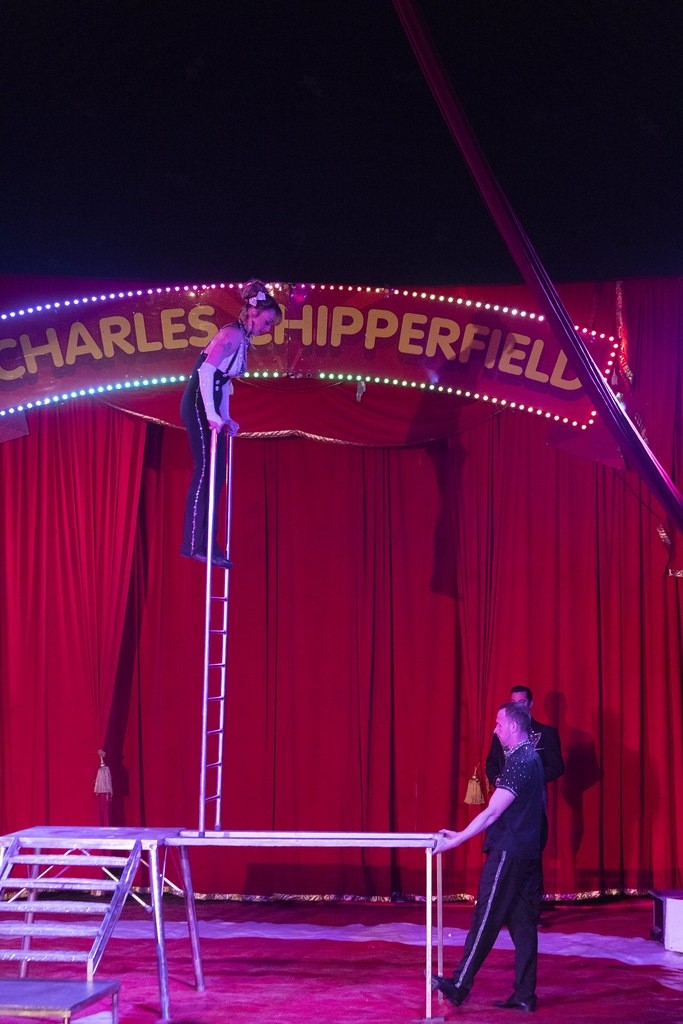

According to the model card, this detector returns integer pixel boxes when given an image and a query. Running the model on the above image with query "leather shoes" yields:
[185,551,234,569]
[431,976,469,1006]
[493,993,536,1012]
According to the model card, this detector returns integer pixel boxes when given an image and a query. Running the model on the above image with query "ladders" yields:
[197,426,232,839]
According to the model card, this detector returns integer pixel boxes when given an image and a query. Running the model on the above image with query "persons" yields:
[484,685,564,857]
[423,702,547,1013]
[179,280,283,569]
